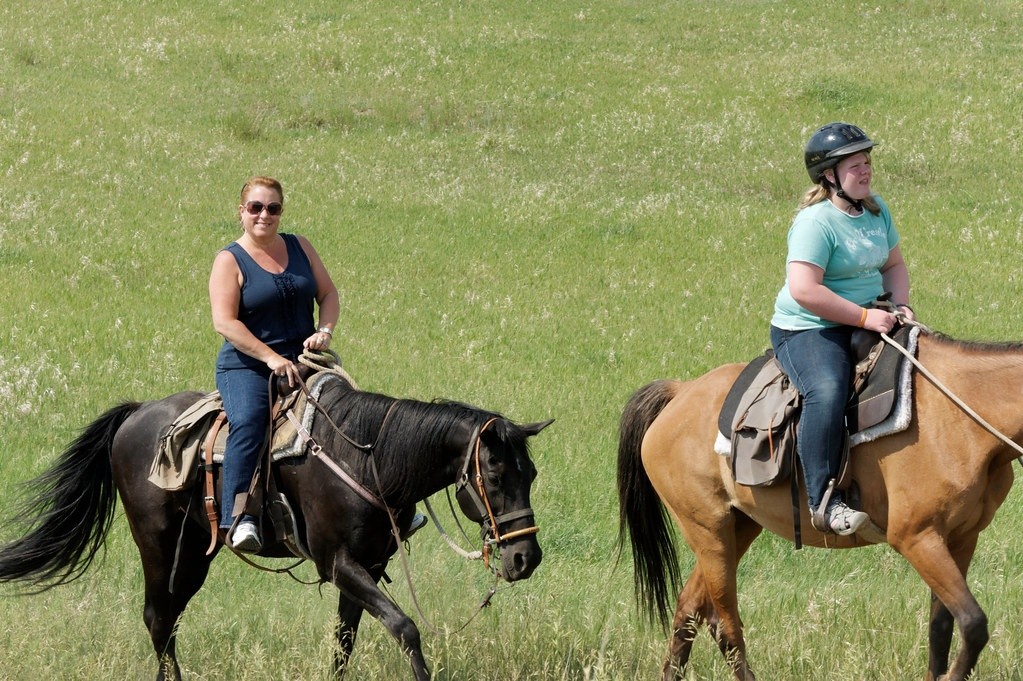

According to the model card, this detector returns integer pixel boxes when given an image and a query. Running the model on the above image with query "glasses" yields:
[244,200,285,216]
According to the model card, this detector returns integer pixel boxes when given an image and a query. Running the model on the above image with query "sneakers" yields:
[809,502,868,536]
[408,513,423,532]
[231,523,262,552]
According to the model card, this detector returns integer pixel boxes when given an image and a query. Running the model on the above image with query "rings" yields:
[322,343,327,347]
[280,372,285,376]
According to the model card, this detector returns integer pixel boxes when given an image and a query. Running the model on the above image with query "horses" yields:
[0,368,555,681]
[615,321,1022,681]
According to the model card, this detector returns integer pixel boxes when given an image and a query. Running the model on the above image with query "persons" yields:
[768,123,914,534]
[210,177,428,553]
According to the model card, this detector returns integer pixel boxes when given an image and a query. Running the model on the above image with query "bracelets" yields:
[858,308,867,327]
[315,327,333,339]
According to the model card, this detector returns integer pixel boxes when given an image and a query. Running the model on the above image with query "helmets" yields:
[805,123,879,184]
